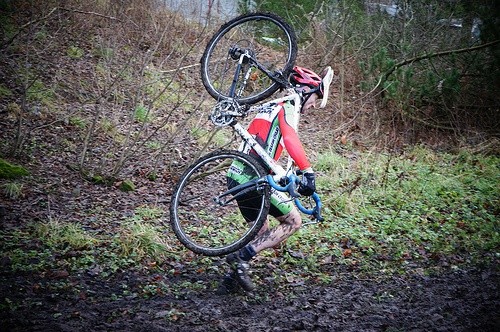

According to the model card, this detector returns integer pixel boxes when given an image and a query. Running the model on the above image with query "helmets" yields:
[289,66,324,98]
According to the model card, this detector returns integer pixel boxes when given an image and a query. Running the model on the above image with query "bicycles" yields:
[169,12,334,255]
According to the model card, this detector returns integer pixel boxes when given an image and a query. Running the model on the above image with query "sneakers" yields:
[226,254,254,292]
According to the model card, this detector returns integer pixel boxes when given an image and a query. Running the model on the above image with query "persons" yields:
[217,66,334,294]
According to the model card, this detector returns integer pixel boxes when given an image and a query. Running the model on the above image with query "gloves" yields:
[297,173,315,196]
